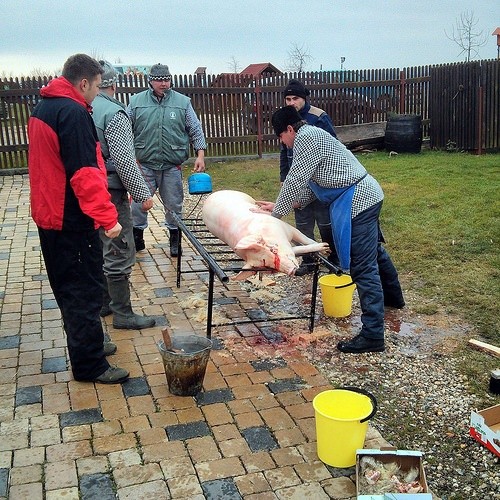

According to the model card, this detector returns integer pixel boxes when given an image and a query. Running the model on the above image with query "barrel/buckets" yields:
[157,334,213,397]
[318,274,357,318]
[312,387,378,469]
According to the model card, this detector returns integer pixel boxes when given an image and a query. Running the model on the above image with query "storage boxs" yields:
[355,448,433,500]
[470,404,500,457]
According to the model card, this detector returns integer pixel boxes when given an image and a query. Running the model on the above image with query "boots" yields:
[168,229,183,256]
[100,276,112,317]
[132,227,146,251]
[106,276,155,329]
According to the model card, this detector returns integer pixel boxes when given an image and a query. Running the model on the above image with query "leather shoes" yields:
[383,298,406,308]
[338,334,384,353]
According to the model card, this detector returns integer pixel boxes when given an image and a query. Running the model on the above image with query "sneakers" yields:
[86,365,130,384]
[103,343,117,356]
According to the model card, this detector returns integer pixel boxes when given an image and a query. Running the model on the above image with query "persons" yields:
[27,54,130,384]
[90,61,156,329]
[279,79,340,276]
[250,105,405,353]
[126,64,206,257]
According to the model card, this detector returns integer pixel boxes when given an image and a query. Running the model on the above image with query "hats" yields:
[148,64,171,81]
[95,60,117,88]
[283,80,305,100]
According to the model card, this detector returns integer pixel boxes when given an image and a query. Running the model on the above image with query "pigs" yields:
[201,188,331,283]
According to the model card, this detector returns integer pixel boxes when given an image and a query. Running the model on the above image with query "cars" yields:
[317,71,395,113]
[243,74,371,138]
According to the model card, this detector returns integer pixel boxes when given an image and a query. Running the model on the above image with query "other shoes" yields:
[295,265,319,275]
